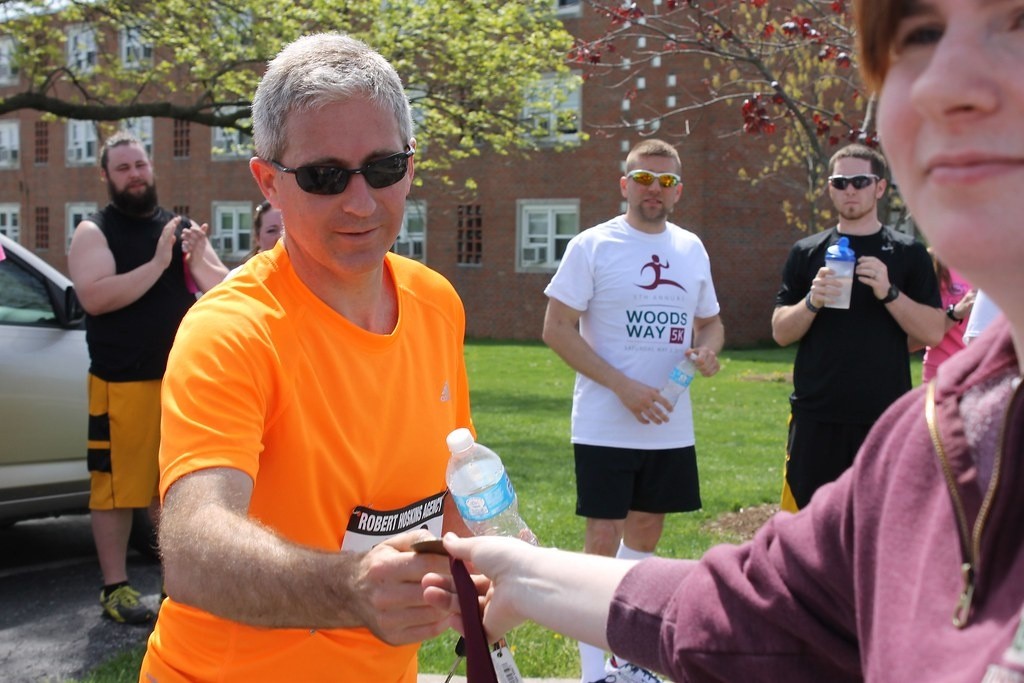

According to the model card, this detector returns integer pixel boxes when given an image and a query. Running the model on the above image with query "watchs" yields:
[879,285,900,304]
[944,303,963,325]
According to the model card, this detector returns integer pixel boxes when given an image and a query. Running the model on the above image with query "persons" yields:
[67,132,234,626]
[422,0,1024,682]
[772,143,1003,515]
[250,201,286,257]
[137,33,476,683]
[540,139,725,683]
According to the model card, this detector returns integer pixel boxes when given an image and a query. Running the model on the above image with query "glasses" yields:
[627,169,681,187]
[828,174,880,190]
[268,142,416,195]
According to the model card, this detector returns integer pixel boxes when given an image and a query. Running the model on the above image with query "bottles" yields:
[823,238,855,311]
[648,352,696,417]
[444,427,542,548]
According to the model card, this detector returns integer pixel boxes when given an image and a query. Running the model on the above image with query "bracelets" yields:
[805,292,819,314]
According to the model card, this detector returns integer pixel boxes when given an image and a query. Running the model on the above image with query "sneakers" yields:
[100,586,158,624]
[588,658,663,683]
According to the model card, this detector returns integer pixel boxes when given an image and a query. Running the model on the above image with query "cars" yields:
[0,230,97,534]
